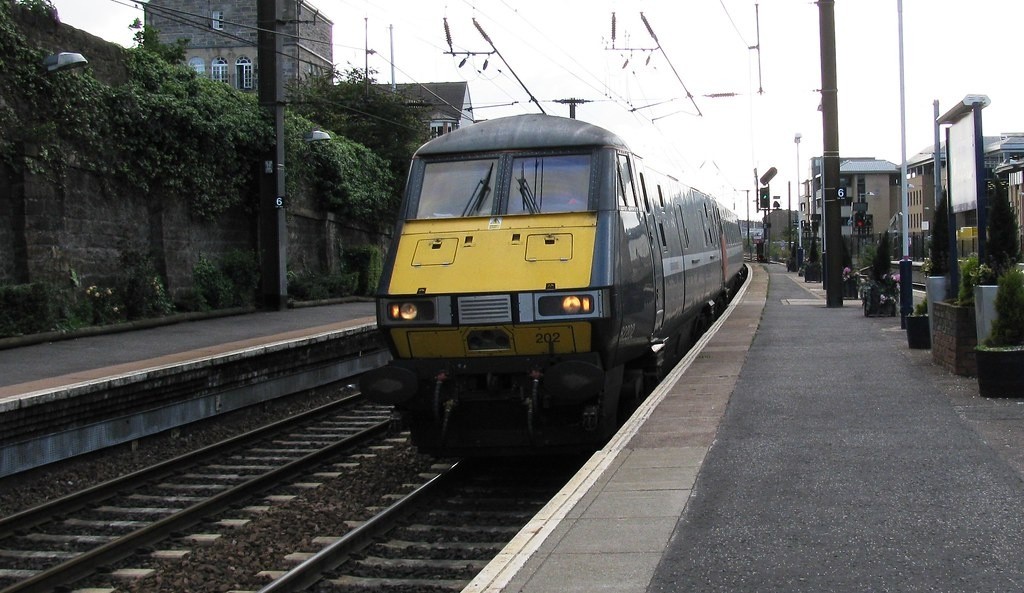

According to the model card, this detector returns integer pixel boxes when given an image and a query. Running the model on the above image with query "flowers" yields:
[861,229,901,305]
[842,235,859,283]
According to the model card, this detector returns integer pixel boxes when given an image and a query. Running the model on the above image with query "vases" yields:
[843,279,859,301]
[861,286,899,319]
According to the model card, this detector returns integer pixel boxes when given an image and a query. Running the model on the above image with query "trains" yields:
[374,109,746,459]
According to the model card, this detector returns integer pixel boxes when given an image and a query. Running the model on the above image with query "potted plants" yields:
[904,293,930,349]
[973,266,1024,398]
[922,186,950,351]
[805,236,822,283]
[970,175,1020,346]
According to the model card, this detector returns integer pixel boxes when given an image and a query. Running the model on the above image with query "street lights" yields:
[733,189,750,248]
[794,133,804,270]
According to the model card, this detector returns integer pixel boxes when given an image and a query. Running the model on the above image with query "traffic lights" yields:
[853,212,865,230]
[760,187,769,208]
[865,214,873,228]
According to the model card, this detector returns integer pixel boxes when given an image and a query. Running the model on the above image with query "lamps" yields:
[290,131,330,142]
[25,52,89,80]
